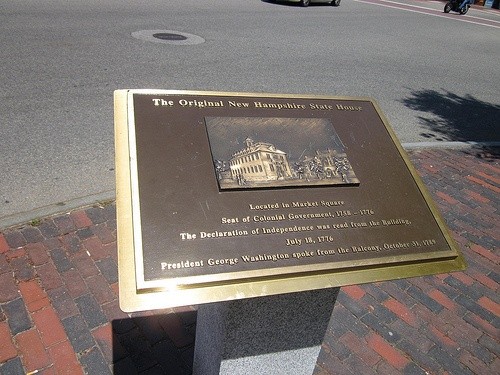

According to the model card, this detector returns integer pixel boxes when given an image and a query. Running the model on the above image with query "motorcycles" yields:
[443,0,472,14]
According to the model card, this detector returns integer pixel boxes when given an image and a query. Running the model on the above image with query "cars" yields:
[265,0,342,6]
